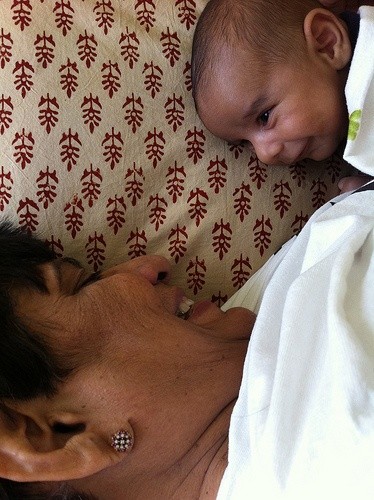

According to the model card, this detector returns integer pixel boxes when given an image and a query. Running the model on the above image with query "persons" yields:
[191,0,374,194]
[0,182,374,500]
